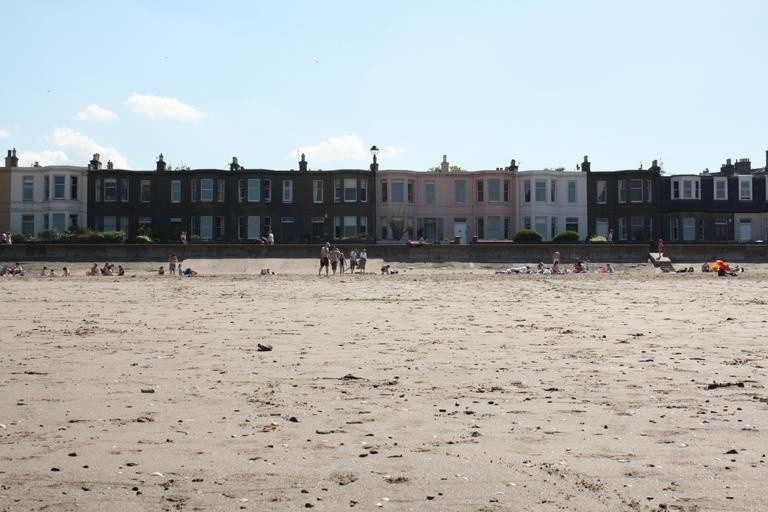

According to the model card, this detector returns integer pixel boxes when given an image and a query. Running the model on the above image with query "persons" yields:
[702,260,711,272]
[318,242,367,276]
[159,254,197,277]
[495,250,615,274]
[0,263,71,277]
[86,262,124,276]
[180,231,187,244]
[718,267,737,276]
[268,230,274,244]
[657,239,664,257]
[608,229,614,244]
[1,232,7,243]
[6,233,12,244]
[381,265,390,274]
